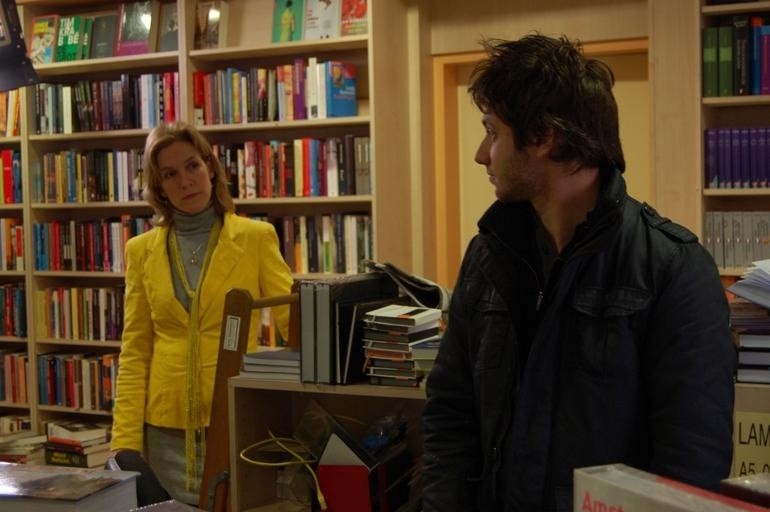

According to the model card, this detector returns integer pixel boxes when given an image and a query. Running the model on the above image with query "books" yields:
[304,0,340,39]
[0,217,24,273]
[271,0,303,42]
[729,263,770,386]
[159,3,177,52]
[89,14,118,58]
[2,283,29,339]
[32,285,123,341]
[2,83,21,137]
[2,352,31,404]
[705,209,770,271]
[47,414,111,462]
[703,16,769,99]
[342,1,367,36]
[192,0,219,51]
[31,71,182,134]
[276,213,371,274]
[364,263,445,386]
[241,346,295,377]
[55,12,92,62]
[707,124,770,190]
[31,146,149,202]
[1,415,47,461]
[116,2,153,54]
[297,277,365,385]
[209,134,374,200]
[36,352,115,410]
[28,14,56,67]
[0,150,24,204]
[32,218,155,272]
[190,58,356,123]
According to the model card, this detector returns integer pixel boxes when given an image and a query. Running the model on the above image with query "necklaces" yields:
[176,228,208,264]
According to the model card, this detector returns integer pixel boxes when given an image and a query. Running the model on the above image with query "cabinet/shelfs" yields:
[0,0,377,467]
[228,375,427,512]
[699,1,770,276]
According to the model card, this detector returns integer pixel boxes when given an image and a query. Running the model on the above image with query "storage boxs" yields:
[310,456,411,511]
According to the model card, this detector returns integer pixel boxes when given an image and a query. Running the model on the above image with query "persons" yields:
[113,125,293,509]
[415,34,737,510]
